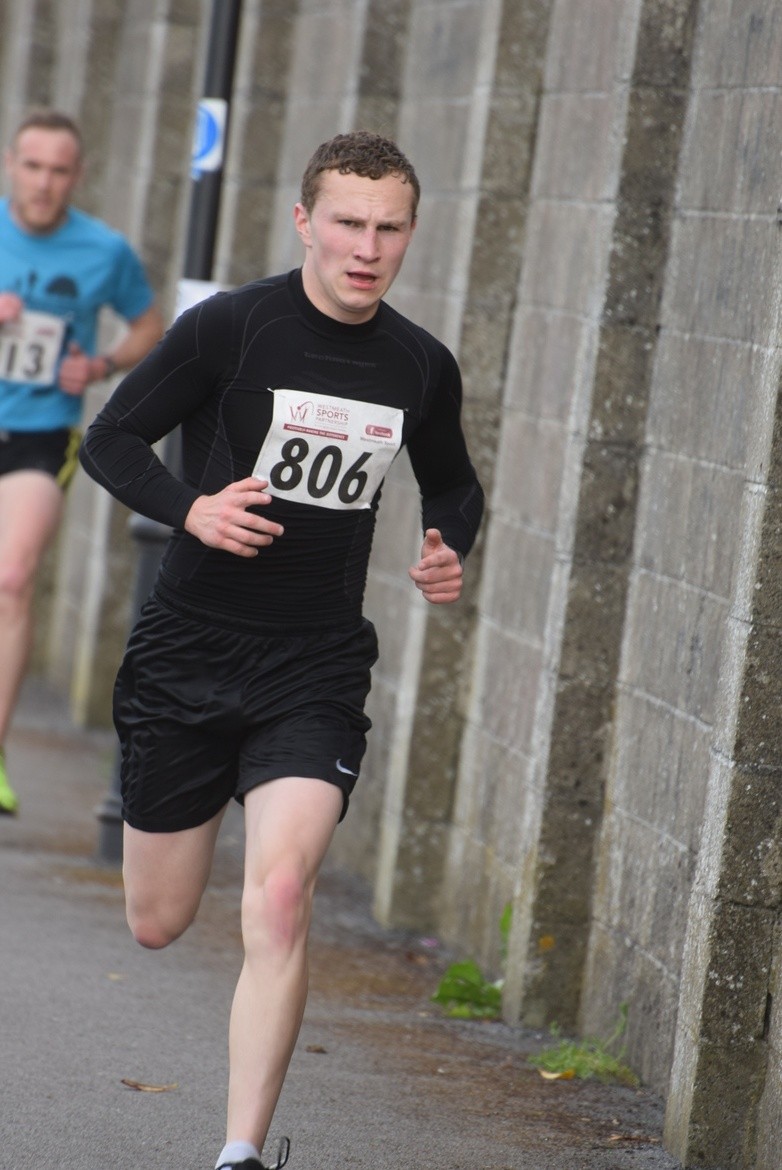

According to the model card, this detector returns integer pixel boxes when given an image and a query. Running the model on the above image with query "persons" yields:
[1,112,166,815]
[75,134,488,1170]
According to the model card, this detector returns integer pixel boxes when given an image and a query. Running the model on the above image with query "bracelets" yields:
[101,354,116,381]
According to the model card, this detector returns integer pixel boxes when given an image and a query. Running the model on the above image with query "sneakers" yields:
[215,1136,292,1170]
[0,747,18,817]
